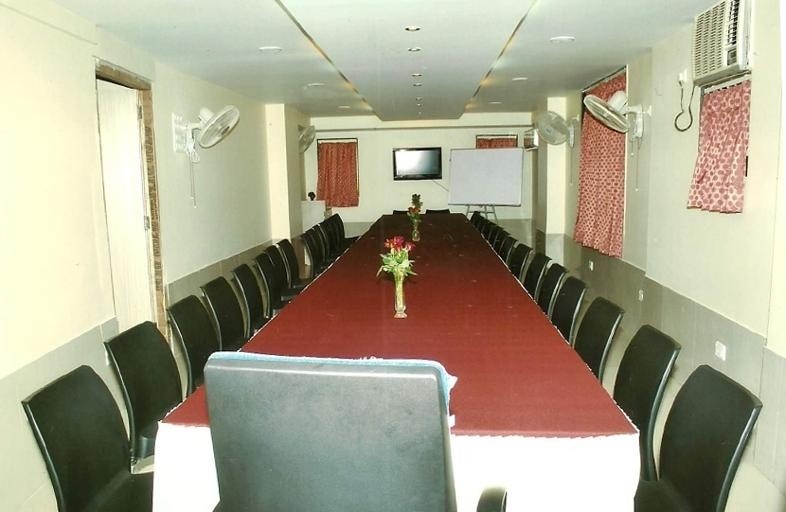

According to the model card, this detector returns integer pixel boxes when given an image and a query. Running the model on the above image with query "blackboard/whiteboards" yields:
[448,147,524,207]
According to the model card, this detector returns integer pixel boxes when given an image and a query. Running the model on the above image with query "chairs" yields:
[22,366,153,512]
[538,263,569,317]
[393,210,409,214]
[469,211,510,253]
[203,350,508,512]
[199,275,250,352]
[633,366,763,512]
[508,242,534,282]
[574,296,626,386]
[499,237,518,264]
[552,276,589,345]
[276,238,313,291]
[265,245,303,301]
[256,253,288,318]
[300,213,359,279]
[104,321,183,466]
[233,264,270,340]
[426,209,450,214]
[166,295,220,398]
[614,324,681,483]
[523,252,552,299]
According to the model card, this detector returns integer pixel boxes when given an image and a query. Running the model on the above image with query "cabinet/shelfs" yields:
[302,200,333,233]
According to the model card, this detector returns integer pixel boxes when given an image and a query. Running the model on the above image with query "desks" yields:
[151,214,640,512]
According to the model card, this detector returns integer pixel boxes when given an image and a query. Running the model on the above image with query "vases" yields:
[412,220,422,241]
[393,273,407,319]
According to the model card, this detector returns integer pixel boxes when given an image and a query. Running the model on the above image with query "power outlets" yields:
[679,70,687,82]
[576,115,579,121]
[648,105,652,117]
[589,261,593,271]
[715,340,726,362]
[638,289,643,301]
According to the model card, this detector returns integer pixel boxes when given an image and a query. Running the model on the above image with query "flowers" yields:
[407,207,421,227]
[377,235,418,281]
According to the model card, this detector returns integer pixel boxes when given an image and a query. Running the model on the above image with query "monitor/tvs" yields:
[392,146,443,183]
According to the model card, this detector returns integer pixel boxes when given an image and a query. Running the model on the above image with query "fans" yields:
[583,90,643,141]
[171,105,240,154]
[298,126,315,155]
[535,111,574,148]
